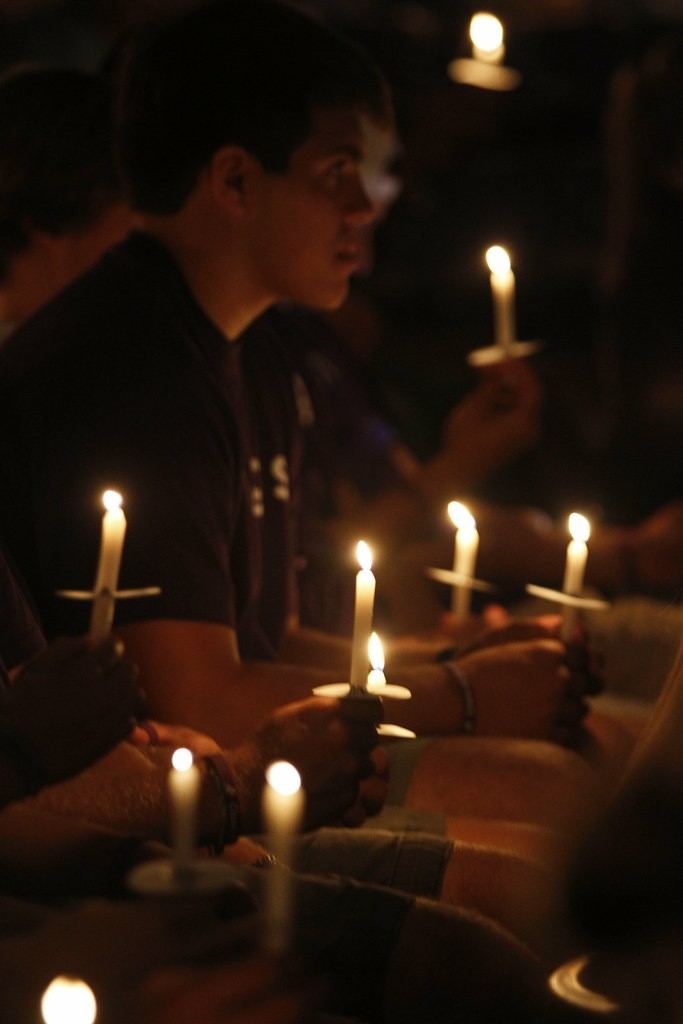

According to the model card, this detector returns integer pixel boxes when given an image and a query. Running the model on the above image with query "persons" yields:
[0,1,610,841]
[0,64,682,1024]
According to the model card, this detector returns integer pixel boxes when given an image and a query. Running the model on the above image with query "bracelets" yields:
[444,660,478,736]
[197,755,238,842]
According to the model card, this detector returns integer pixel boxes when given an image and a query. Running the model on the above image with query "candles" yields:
[169,771,204,873]
[495,275,518,345]
[452,527,480,619]
[350,570,376,694]
[562,543,588,644]
[267,798,305,963]
[87,507,125,636]
